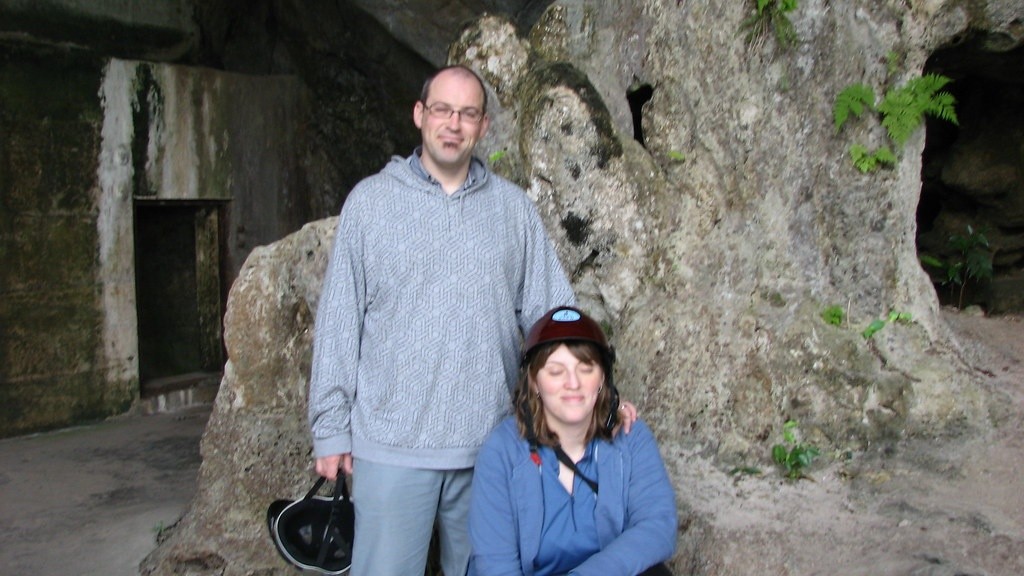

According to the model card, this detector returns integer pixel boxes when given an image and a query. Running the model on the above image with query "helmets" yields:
[266,494,355,575]
[520,302,612,357]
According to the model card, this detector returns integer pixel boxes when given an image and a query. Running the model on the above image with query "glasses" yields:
[420,101,486,125]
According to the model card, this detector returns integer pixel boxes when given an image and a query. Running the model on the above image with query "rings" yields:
[621,404,627,410]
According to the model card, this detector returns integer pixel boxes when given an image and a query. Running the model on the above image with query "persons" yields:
[308,65,635,576]
[468,306,680,576]
